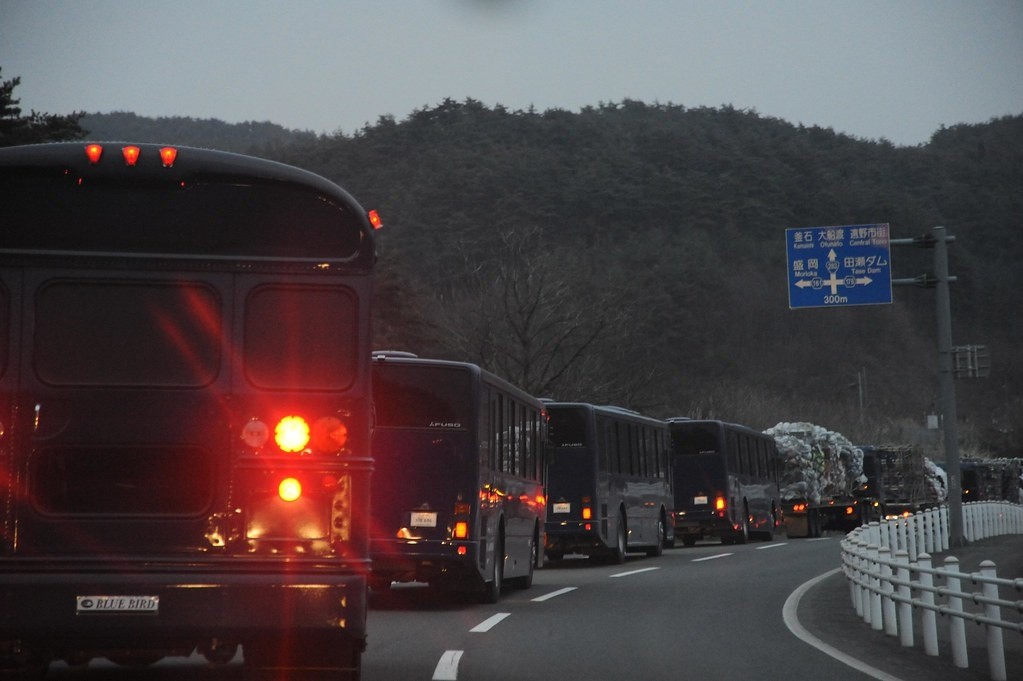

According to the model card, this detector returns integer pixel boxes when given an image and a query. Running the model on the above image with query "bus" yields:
[0,142,383,681]
[536,398,675,564]
[366,350,554,609]
[664,417,781,549]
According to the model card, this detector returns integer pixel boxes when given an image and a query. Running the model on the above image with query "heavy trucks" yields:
[762,422,1023,538]
